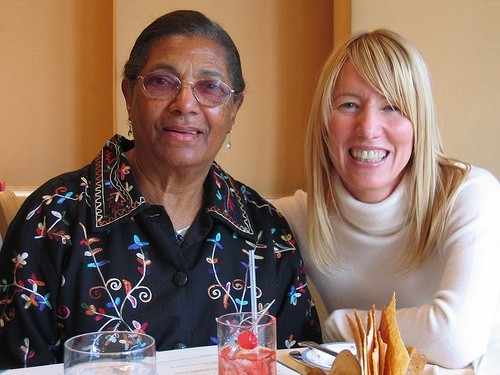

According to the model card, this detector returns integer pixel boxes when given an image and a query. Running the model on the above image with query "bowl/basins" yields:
[301,341,364,375]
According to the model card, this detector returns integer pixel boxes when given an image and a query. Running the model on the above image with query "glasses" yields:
[133,71,238,107]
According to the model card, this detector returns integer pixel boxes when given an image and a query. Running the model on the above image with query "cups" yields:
[217,312,277,375]
[63,331,157,375]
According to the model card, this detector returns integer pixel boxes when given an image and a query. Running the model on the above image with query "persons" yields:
[1,9,326,370]
[261,30,500,369]
